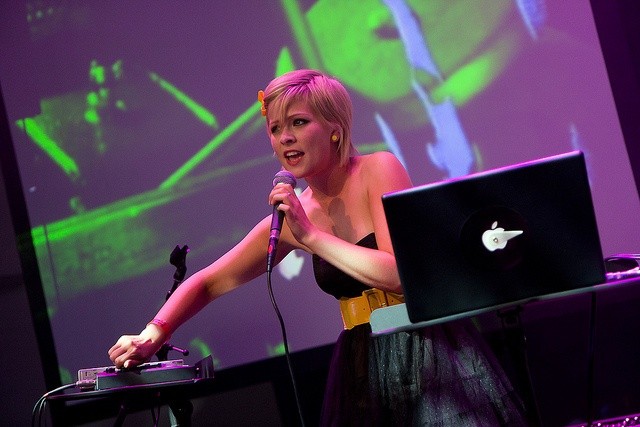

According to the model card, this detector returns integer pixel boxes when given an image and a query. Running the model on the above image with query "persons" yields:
[108,68,529,426]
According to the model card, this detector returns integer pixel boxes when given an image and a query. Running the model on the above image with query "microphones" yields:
[266,170,297,271]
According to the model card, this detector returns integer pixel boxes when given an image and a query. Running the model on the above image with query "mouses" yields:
[605,256,638,272]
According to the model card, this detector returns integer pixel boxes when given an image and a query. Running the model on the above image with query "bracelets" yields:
[147,319,171,337]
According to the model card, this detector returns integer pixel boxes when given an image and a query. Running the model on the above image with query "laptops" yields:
[381,150,607,324]
